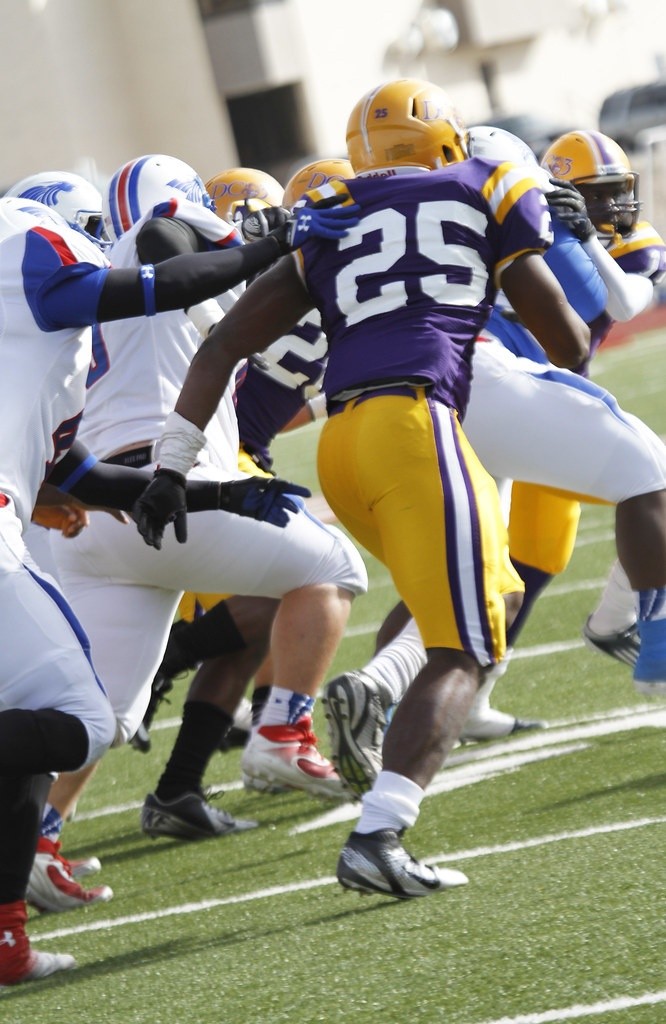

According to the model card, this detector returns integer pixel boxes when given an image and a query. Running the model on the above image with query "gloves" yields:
[134,468,190,552]
[544,178,596,242]
[268,194,360,254]
[218,475,311,529]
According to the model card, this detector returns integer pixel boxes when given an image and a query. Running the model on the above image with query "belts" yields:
[324,382,424,416]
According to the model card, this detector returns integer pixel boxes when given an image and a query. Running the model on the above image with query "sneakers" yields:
[129,670,189,754]
[0,899,76,986]
[238,715,356,800]
[142,785,259,842]
[337,826,470,899]
[321,673,388,798]
[26,837,113,914]
[456,704,549,746]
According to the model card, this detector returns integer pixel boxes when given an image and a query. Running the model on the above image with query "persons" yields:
[0,81,666,979]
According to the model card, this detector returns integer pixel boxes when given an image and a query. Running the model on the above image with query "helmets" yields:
[465,126,536,162]
[100,154,218,242]
[345,77,470,175]
[541,129,634,185]
[281,158,356,213]
[203,168,285,224]
[1,170,105,239]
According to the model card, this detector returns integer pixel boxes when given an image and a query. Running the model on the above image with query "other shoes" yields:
[629,618,666,696]
[220,697,254,752]
[582,614,642,667]
[65,855,102,879]
